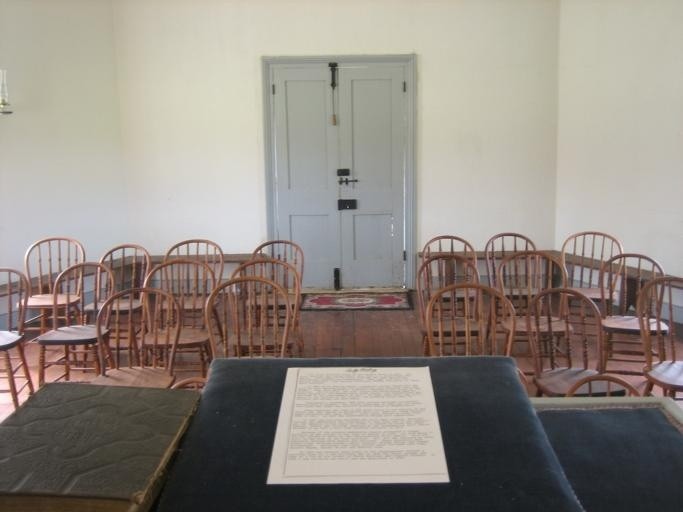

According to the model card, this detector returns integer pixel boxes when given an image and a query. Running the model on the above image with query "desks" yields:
[1,380,682,512]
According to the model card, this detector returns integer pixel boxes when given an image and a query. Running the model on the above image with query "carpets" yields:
[301,293,415,311]
